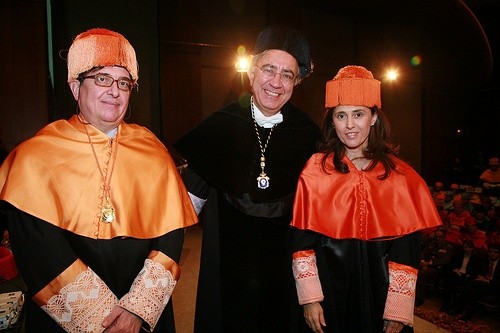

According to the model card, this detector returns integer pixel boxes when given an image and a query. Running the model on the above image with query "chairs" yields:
[450,249,500,315]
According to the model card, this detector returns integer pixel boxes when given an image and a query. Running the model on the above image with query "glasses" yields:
[75,73,135,91]
[255,63,298,83]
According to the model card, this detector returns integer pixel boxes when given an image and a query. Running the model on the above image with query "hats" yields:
[66,27,139,86]
[324,65,382,109]
[253,23,314,78]
[433,156,500,224]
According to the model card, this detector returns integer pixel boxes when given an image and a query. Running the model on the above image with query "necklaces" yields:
[251,94,277,189]
[79,112,120,223]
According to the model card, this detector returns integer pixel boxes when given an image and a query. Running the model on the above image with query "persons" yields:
[431,158,500,321]
[1,28,199,332]
[288,66,444,333]
[170,25,330,333]
